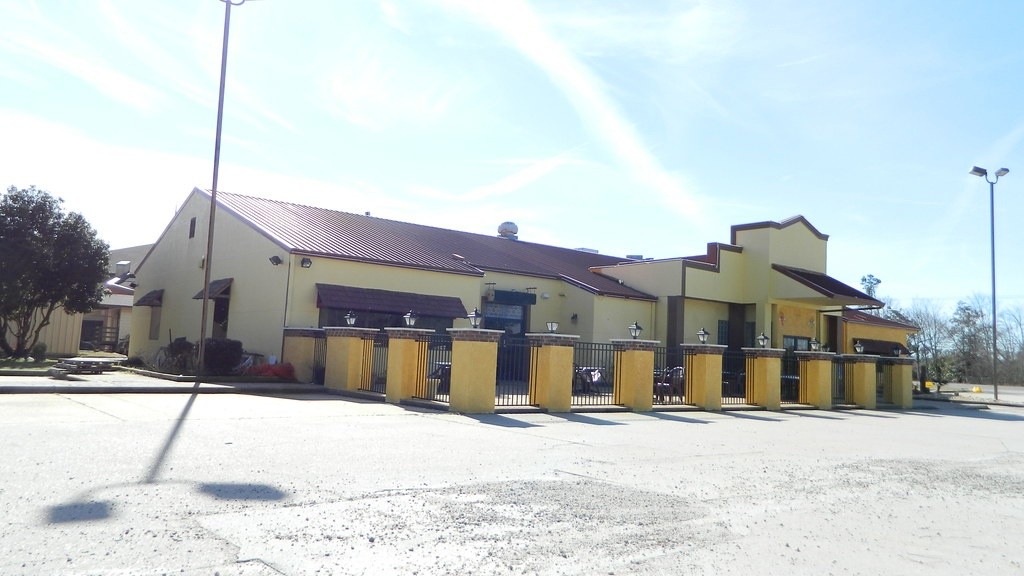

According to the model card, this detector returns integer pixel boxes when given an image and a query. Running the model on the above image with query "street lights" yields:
[968,165,1010,401]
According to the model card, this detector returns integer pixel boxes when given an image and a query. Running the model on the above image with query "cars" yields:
[571,365,799,401]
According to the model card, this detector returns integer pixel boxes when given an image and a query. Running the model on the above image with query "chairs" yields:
[427,363,685,405]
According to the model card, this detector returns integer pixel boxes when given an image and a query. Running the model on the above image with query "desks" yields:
[654,369,671,373]
[574,367,605,370]
[433,362,451,366]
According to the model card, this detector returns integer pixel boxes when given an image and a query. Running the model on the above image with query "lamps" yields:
[403,310,419,328]
[809,337,819,351]
[756,332,769,348]
[467,307,483,328]
[485,283,496,302]
[545,321,559,332]
[892,344,902,356]
[269,256,282,265]
[344,309,359,326]
[302,257,312,268]
[697,327,710,344]
[627,321,643,339]
[853,340,864,353]
